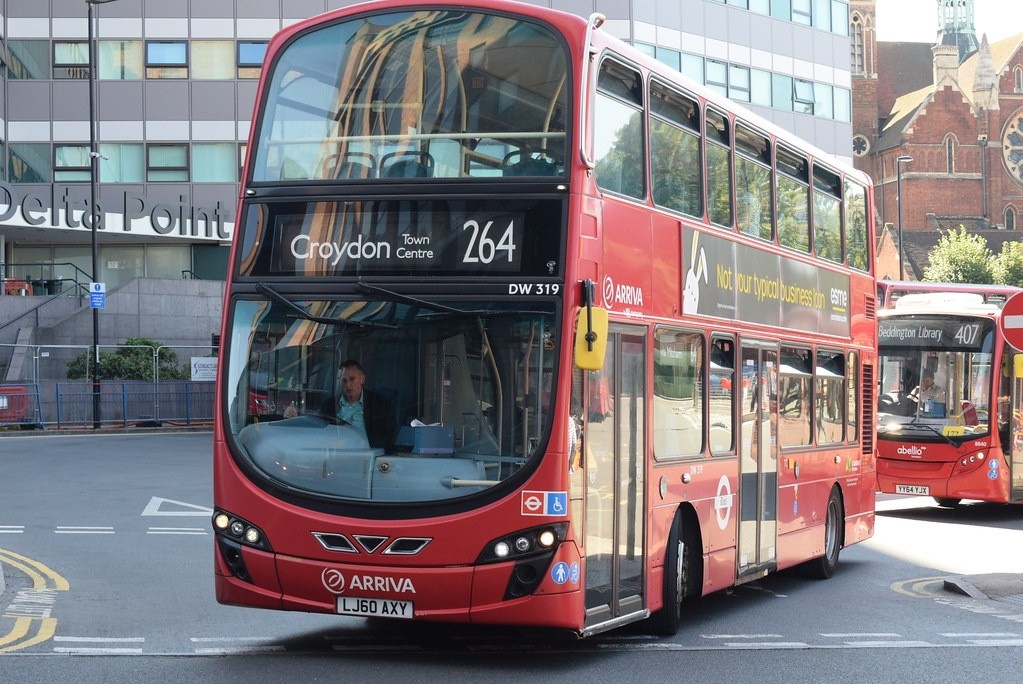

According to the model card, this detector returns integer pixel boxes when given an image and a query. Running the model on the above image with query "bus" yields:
[872,278,1023,512]
[207,0,879,644]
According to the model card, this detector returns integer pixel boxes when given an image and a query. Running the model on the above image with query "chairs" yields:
[321,148,762,239]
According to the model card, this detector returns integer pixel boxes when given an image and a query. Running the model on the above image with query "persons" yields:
[761,377,767,407]
[738,179,762,238]
[743,378,749,399]
[720,376,732,394]
[905,368,947,404]
[279,358,397,457]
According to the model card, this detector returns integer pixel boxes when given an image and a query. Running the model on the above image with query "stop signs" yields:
[999,289,1023,354]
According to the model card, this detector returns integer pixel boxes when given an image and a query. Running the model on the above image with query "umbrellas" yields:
[815,366,844,380]
[780,364,814,379]
[707,360,734,375]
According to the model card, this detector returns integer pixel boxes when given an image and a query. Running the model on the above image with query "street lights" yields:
[895,154,915,281]
[80,0,125,429]
